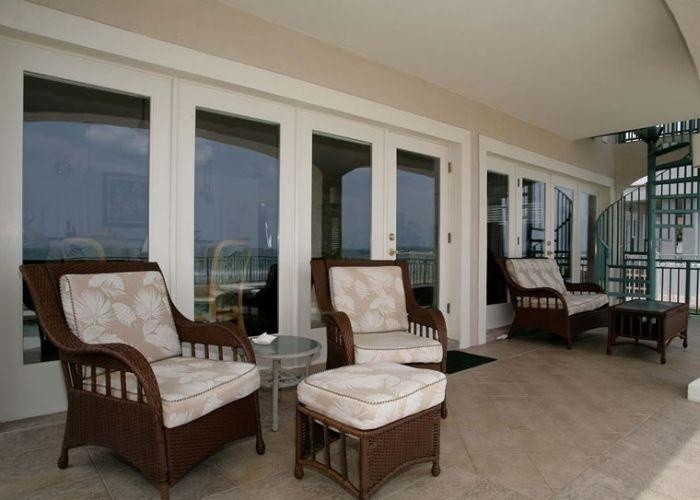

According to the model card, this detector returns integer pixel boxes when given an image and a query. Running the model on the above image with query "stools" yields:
[294,361,448,500]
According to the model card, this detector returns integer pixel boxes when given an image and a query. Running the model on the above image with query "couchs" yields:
[494,256,610,350]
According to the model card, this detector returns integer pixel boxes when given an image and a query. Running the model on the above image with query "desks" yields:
[237,335,321,431]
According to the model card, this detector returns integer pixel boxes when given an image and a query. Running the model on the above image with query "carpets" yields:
[446,351,497,374]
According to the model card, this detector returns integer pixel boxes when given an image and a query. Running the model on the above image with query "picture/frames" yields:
[101,172,148,228]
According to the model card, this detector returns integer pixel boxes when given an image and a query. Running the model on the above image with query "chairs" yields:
[310,258,448,418]
[58,237,107,260]
[19,262,266,500]
[195,240,254,336]
